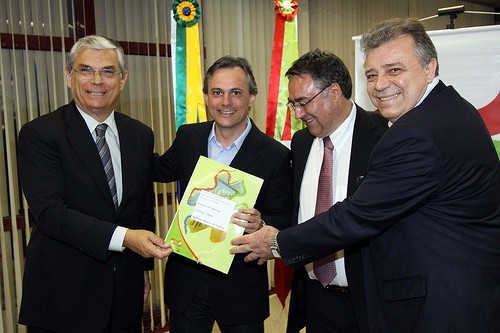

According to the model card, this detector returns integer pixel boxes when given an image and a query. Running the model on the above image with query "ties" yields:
[312,137,338,288]
[96,122,119,208]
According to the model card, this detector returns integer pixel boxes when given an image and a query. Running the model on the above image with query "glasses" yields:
[69,66,123,80]
[286,80,337,112]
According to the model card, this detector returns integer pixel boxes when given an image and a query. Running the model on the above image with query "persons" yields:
[284,48,390,333]
[150,56,292,333]
[229,16,500,333]
[16,35,173,333]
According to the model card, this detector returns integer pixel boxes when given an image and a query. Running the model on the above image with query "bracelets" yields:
[260,219,266,227]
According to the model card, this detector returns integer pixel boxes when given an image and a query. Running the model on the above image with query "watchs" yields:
[270,230,283,258]
